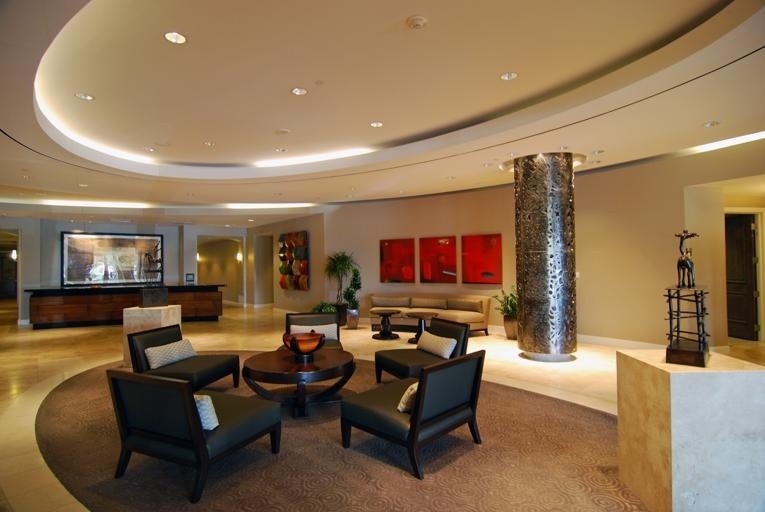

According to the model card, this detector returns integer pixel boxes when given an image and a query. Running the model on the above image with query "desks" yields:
[23,285,227,329]
[122,304,182,362]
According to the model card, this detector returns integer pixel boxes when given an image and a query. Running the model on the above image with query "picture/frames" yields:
[186,273,194,281]
[61,230,164,287]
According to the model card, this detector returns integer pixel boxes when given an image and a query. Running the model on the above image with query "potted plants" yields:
[323,248,361,326]
[343,267,362,329]
[491,284,517,340]
[137,241,169,307]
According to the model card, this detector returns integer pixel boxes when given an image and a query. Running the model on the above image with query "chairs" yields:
[340,350,485,481]
[126,323,239,393]
[286,311,343,350]
[375,317,470,385]
[106,367,281,504]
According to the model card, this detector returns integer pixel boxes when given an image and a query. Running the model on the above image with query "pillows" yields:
[395,380,419,413]
[370,296,410,306]
[417,330,457,360]
[448,298,483,313]
[193,394,221,431]
[290,323,339,341]
[409,296,448,310]
[144,338,198,371]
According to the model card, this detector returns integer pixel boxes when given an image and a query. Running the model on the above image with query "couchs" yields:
[368,292,492,336]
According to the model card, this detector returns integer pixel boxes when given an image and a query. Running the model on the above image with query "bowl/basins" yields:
[283,332,322,353]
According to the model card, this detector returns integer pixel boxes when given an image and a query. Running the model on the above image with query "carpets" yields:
[35,350,651,512]
